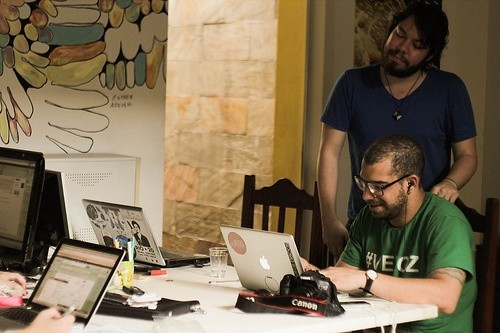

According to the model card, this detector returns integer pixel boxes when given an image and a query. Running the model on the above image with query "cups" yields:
[113,260,134,290]
[209,247,228,278]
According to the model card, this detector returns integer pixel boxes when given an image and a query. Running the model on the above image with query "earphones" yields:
[407,183,413,191]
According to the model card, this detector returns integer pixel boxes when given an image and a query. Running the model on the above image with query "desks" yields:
[84,263,437,333]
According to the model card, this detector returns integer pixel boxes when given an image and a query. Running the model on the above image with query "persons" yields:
[131,221,150,248]
[299,133,477,333]
[317,3,486,263]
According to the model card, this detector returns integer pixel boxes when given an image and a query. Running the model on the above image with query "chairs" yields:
[241,175,328,272]
[450,196,500,333]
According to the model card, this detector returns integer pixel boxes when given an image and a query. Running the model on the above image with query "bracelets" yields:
[445,177,459,189]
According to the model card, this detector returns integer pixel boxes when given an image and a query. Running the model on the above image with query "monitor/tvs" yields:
[0,146,45,275]
[30,170,70,266]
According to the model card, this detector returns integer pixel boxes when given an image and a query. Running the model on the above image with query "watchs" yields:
[364,269,378,291]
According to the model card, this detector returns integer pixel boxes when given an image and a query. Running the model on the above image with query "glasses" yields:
[354,174,412,196]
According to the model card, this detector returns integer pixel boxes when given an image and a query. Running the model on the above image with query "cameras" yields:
[279,270,337,302]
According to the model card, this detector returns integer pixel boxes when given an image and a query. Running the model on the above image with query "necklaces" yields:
[383,67,423,121]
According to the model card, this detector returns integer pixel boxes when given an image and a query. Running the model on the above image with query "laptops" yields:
[81,199,210,268]
[219,225,367,302]
[0,238,127,333]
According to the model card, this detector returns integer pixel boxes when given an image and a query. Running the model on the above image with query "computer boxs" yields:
[40,152,141,244]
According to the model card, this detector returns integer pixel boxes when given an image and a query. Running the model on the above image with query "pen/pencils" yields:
[142,270,167,275]
[209,280,239,283]
[60,306,74,317]
